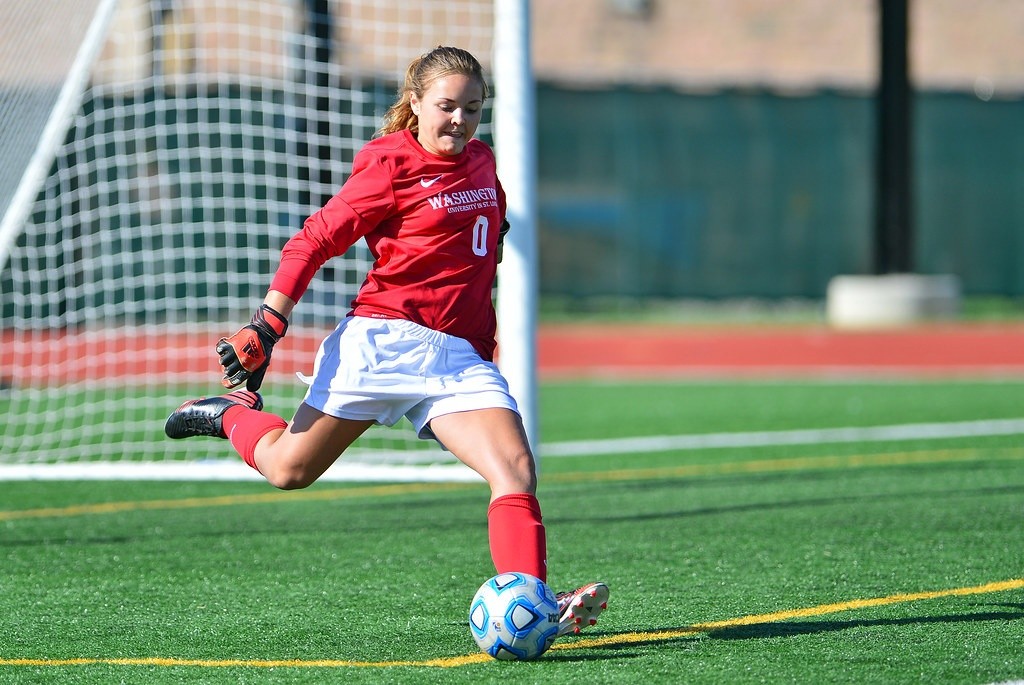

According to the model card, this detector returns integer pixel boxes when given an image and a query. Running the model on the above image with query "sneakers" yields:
[555,583,609,636]
[164,387,263,439]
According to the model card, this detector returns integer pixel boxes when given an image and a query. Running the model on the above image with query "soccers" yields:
[466,571,562,663]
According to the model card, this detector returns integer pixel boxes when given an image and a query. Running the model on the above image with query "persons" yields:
[164,49,611,637]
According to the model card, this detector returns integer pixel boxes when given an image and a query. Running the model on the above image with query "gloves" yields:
[215,303,289,394]
